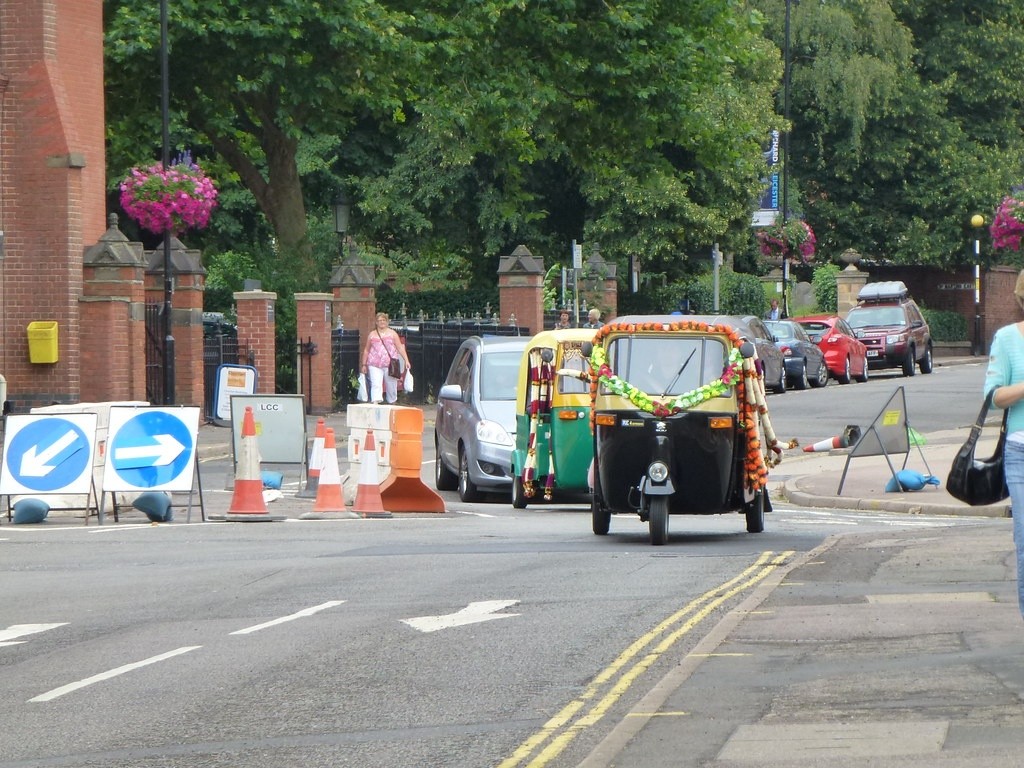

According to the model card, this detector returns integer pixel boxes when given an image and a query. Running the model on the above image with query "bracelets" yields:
[362,365,366,366]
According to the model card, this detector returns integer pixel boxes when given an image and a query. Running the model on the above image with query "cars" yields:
[736,315,788,394]
[435,334,533,503]
[763,321,830,390]
[786,316,869,384]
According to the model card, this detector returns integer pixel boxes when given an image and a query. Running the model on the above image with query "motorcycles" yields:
[581,316,773,546]
[510,329,598,508]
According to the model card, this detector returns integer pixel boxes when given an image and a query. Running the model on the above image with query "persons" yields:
[362,312,411,405]
[982,267,1024,618]
[584,309,605,329]
[555,311,571,329]
[763,300,783,321]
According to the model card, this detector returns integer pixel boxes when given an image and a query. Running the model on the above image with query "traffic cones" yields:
[206,406,288,521]
[294,418,326,496]
[803,425,861,454]
[298,428,357,518]
[351,431,392,518]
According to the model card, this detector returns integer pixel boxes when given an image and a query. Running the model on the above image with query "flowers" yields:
[755,212,816,265]
[120,149,218,237]
[589,320,799,489]
[990,195,1024,251]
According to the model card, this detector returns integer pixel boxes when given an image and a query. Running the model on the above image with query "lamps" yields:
[332,185,353,258]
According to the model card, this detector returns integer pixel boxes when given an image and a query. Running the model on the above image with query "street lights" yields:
[971,215,985,358]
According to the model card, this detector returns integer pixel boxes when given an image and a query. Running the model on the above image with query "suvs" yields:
[845,282,935,375]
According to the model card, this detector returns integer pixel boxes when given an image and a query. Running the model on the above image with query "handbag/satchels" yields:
[404,368,413,392]
[946,384,1009,506]
[388,358,400,379]
[357,373,369,402]
